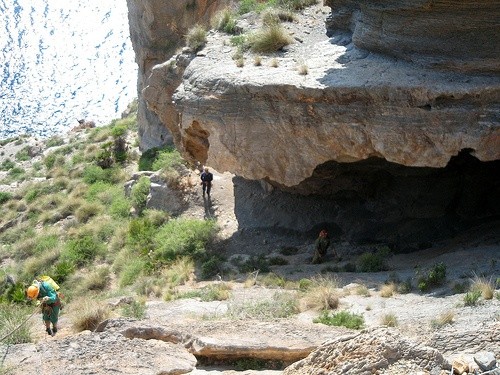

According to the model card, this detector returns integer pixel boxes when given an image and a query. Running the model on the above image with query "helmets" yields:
[320,230,327,236]
[27,284,40,299]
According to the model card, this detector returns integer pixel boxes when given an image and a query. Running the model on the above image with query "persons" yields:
[200,168,213,197]
[27,281,60,335]
[310,229,330,266]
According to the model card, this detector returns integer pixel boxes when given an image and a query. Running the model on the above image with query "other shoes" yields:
[53,327,57,333]
[48,330,52,335]
